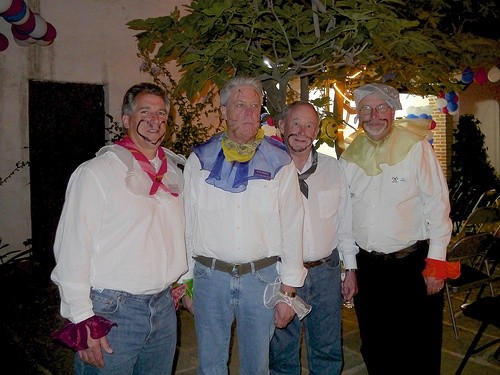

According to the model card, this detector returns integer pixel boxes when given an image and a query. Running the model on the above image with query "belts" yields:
[355,243,417,263]
[278,257,322,269]
[196,255,279,279]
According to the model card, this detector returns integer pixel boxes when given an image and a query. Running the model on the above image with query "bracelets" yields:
[280,289,296,298]
[178,295,187,306]
[345,269,355,272]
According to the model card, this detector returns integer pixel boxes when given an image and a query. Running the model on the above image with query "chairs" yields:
[444,187,500,375]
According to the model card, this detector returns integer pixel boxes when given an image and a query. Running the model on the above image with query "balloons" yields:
[0,33,9,52]
[0,0,57,47]
[405,113,436,150]
[461,66,474,84]
[473,67,488,86]
[435,91,460,116]
[487,66,500,83]
[317,117,339,142]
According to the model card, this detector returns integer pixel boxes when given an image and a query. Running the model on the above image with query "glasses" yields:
[359,104,391,114]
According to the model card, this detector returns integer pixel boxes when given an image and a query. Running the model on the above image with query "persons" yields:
[176,76,313,375]
[269,100,359,375]
[338,83,461,375]
[51,83,189,375]
